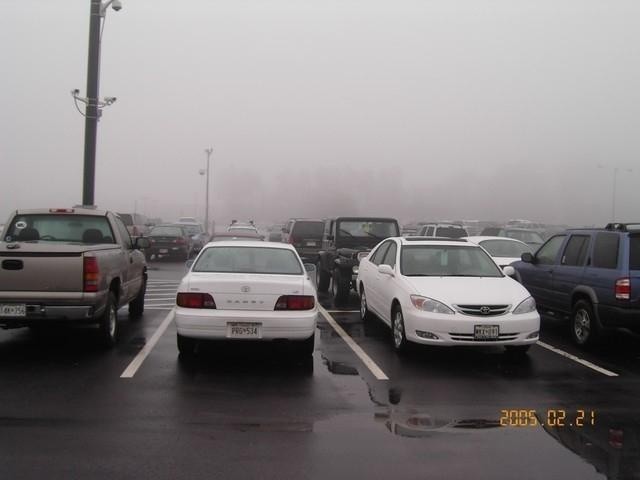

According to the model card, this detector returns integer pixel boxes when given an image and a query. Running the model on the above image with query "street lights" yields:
[83,0,122,206]
[198,149,213,219]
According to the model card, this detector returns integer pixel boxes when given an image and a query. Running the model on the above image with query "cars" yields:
[356,236,540,354]
[209,219,264,241]
[459,236,533,270]
[177,242,317,354]
[122,212,204,260]
[1,207,148,345]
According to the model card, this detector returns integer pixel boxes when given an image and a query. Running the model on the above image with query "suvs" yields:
[479,227,544,252]
[504,222,640,345]
[270,218,401,305]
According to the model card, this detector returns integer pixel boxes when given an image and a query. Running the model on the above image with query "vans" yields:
[419,222,467,239]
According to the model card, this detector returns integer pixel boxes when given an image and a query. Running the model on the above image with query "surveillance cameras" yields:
[112,0,123,11]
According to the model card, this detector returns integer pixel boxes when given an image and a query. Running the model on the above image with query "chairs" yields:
[83,229,104,243]
[17,226,41,241]
